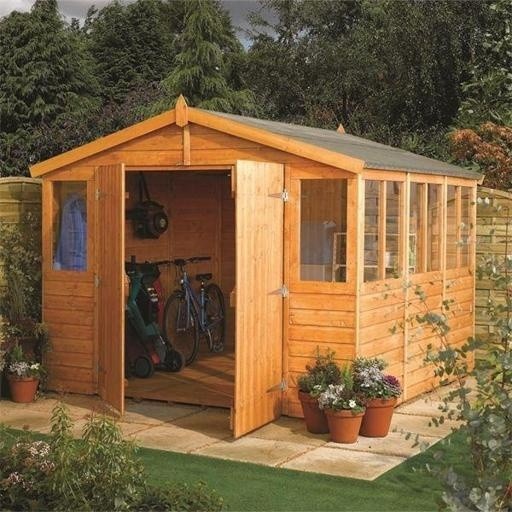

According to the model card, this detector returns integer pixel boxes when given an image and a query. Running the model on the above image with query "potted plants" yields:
[1,245,52,363]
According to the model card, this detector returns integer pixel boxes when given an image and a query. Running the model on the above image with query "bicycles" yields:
[152,256,225,366]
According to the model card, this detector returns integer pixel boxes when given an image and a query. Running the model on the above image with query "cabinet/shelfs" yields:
[331,231,416,284]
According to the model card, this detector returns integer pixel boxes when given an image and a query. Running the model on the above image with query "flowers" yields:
[7,359,50,380]
[317,383,367,416]
[277,347,342,394]
[351,354,404,400]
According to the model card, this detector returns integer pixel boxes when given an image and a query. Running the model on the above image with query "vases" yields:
[321,407,368,445]
[2,375,40,403]
[356,394,398,439]
[297,389,328,435]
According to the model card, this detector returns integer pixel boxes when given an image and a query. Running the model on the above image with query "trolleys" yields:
[123,255,185,379]
